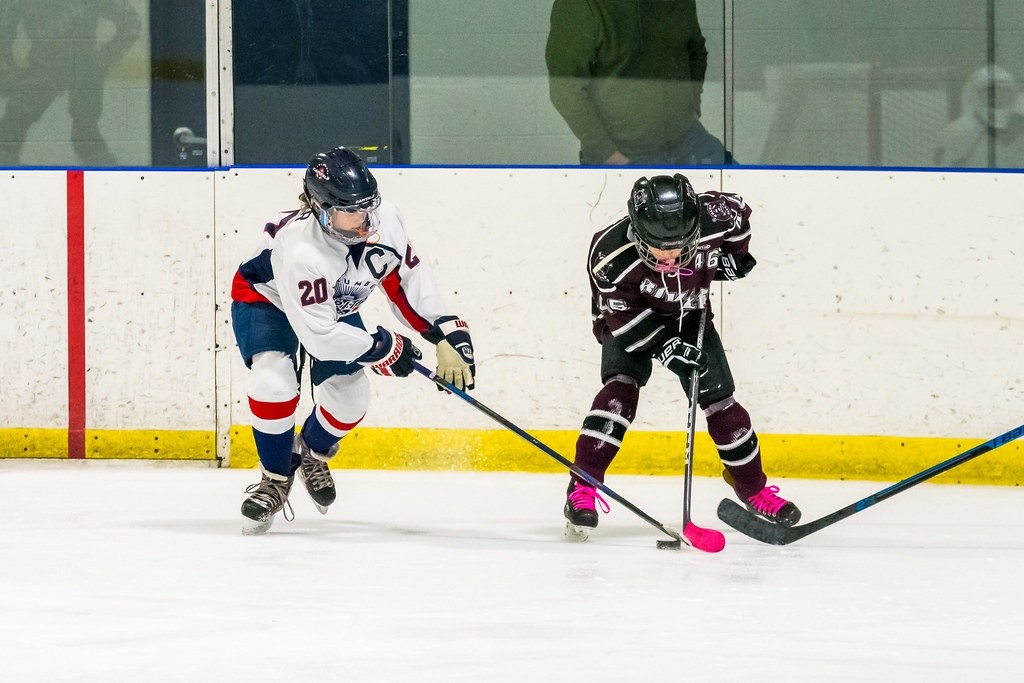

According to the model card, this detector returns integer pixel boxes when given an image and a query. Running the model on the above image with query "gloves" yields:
[653,337,710,378]
[357,327,422,378]
[711,253,757,281]
[420,314,474,394]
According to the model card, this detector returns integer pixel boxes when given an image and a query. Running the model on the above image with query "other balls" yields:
[656,539,681,550]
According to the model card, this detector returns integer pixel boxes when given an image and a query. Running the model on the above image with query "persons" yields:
[544,0,740,168]
[229,150,475,532]
[563,170,802,542]
[936,65,1024,167]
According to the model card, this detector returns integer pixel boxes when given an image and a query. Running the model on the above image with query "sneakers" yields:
[241,452,301,534]
[292,416,339,514]
[564,479,610,543]
[722,469,801,527]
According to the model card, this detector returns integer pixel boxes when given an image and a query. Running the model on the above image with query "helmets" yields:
[304,145,380,214]
[626,173,701,273]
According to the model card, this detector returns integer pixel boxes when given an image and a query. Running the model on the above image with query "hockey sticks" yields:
[411,361,694,549]
[664,292,726,553]
[717,425,1024,548]
